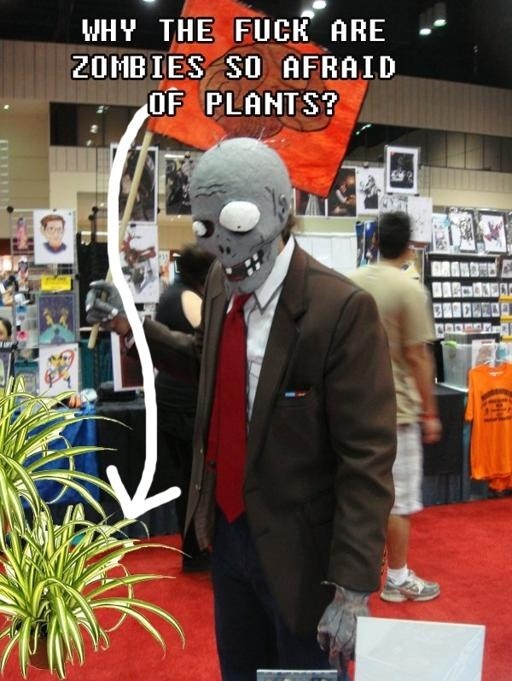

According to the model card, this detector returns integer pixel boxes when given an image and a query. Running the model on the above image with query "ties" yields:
[214,294,253,522]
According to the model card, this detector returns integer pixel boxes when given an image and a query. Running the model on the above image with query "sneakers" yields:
[381,569,441,603]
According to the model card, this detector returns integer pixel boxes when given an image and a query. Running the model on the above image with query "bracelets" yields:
[422,413,440,421]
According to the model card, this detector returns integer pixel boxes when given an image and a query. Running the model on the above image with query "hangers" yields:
[469,343,511,375]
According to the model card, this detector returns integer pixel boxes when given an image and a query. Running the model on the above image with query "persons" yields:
[84,137,399,681]
[41,213,66,253]
[325,173,355,217]
[151,243,214,570]
[345,207,443,603]
[359,175,379,210]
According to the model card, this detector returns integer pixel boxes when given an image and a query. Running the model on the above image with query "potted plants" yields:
[0,372,195,681]
